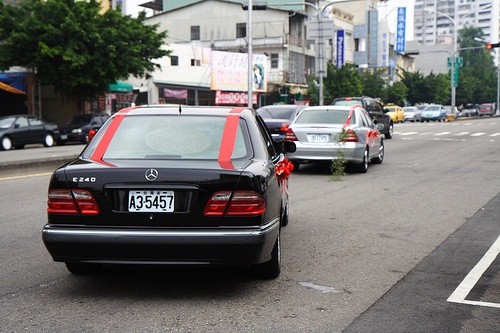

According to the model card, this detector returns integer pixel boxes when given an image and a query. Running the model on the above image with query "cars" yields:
[383,102,405,124]
[44,104,297,280]
[281,105,385,173]
[401,106,420,122]
[0,115,63,151]
[252,104,309,156]
[59,113,111,145]
[454,103,497,118]
[420,105,447,123]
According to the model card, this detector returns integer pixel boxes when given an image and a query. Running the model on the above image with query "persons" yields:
[253,64,265,89]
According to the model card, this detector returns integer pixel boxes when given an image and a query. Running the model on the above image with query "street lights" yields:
[423,4,492,122]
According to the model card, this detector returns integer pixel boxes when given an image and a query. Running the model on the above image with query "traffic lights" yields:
[485,43,500,50]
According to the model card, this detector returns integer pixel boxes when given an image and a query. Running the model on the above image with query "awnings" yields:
[108,81,133,93]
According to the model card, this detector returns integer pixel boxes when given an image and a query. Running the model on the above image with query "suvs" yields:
[331,96,395,140]
